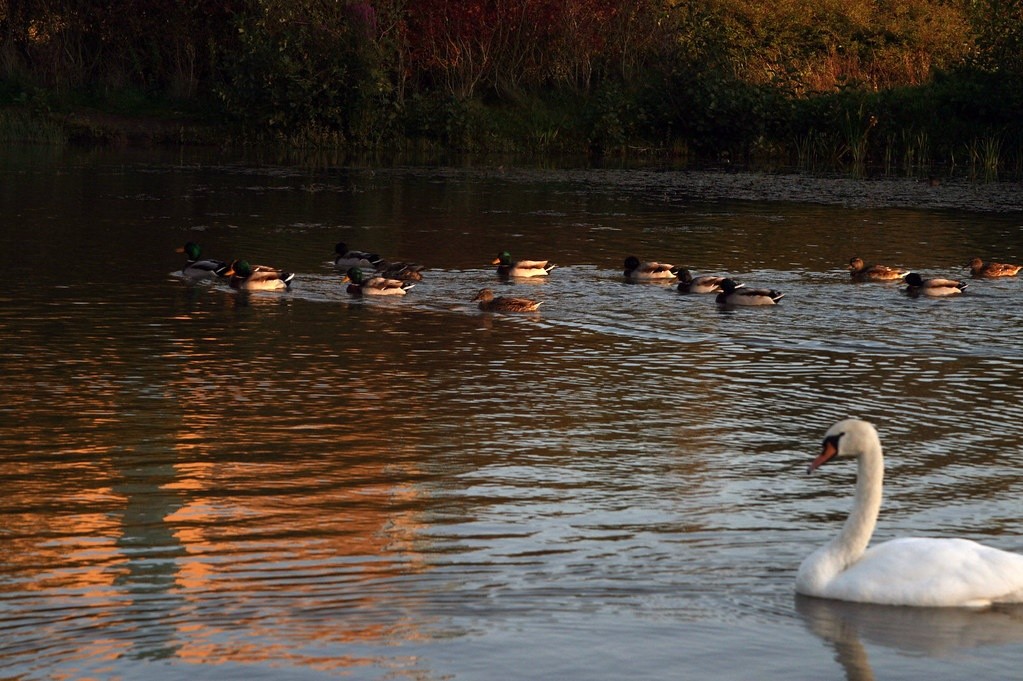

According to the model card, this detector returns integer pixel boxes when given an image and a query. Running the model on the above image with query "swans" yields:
[796,417,1023,608]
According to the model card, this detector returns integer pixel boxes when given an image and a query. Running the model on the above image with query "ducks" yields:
[331,243,385,269]
[846,257,911,282]
[897,273,969,296]
[673,268,745,293]
[374,260,423,281]
[173,242,228,278]
[715,279,786,306]
[470,288,545,312]
[225,258,295,290]
[963,256,1023,278]
[617,257,680,279]
[340,267,417,295]
[491,250,558,277]
